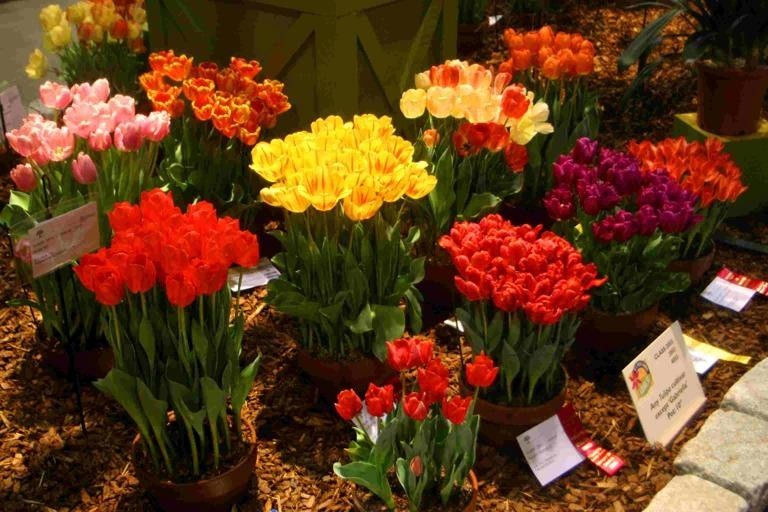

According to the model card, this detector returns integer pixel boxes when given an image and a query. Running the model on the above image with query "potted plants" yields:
[615,2,768,134]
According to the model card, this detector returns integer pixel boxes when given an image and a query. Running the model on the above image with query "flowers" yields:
[623,130,749,259]
[397,59,555,263]
[138,48,293,230]
[544,138,704,315]
[434,211,610,406]
[333,331,502,510]
[70,186,265,476]
[0,76,173,344]
[248,112,438,359]
[482,23,603,203]
[21,0,149,109]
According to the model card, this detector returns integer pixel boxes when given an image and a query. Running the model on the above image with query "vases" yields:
[35,322,115,382]
[667,235,716,283]
[131,409,257,512]
[568,300,661,355]
[498,198,551,231]
[352,463,480,511]
[458,359,569,458]
[412,249,464,310]
[299,348,393,417]
